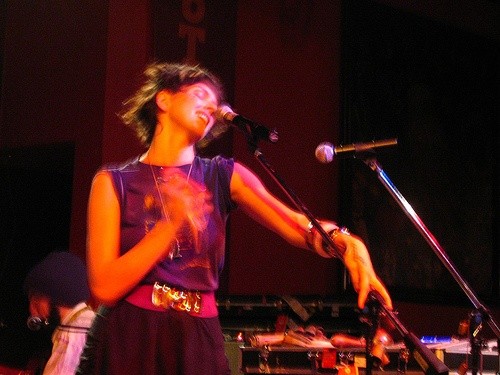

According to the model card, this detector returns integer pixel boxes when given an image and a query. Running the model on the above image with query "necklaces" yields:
[146,149,197,260]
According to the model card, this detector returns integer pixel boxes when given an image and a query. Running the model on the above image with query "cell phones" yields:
[315,137,400,164]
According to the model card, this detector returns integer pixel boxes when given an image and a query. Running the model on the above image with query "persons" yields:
[73,61,397,375]
[23,250,104,375]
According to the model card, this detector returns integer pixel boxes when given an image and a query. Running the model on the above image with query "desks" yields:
[238,340,500,375]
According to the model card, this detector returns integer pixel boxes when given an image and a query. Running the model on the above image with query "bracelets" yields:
[323,226,352,258]
[313,223,339,261]
[306,217,322,249]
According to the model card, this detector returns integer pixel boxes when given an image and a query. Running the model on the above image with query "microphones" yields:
[329,334,367,348]
[216,103,279,143]
